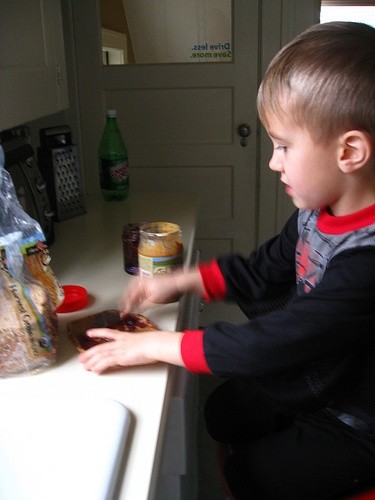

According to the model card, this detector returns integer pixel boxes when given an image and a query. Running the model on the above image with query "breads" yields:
[67,309,160,354]
[0,283,59,378]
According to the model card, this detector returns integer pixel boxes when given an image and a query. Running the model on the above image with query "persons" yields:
[79,21,375,500]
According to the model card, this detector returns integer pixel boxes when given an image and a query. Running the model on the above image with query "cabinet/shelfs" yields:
[0,192,204,500]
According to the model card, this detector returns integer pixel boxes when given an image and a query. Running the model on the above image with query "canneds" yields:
[137,221,185,304]
[121,222,144,275]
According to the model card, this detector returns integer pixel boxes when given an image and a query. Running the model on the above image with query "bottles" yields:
[98,109,129,202]
